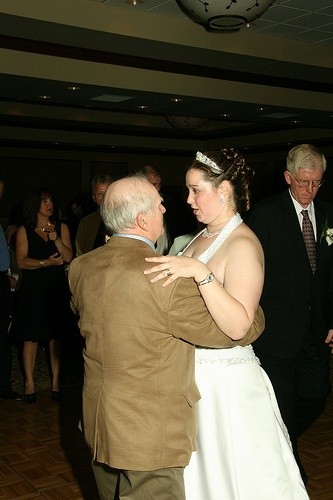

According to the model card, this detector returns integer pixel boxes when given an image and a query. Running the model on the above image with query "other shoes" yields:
[0,389,20,399]
[24,391,36,403]
[53,389,59,402]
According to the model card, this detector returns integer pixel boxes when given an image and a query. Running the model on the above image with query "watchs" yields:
[196,271,215,286]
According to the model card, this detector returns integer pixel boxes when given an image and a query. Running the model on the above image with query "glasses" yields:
[293,173,325,189]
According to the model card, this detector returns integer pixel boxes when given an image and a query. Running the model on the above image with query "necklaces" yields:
[202,227,225,239]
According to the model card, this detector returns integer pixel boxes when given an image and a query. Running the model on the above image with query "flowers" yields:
[319,220,332,246]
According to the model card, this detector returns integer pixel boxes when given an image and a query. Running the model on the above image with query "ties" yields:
[301,210,317,275]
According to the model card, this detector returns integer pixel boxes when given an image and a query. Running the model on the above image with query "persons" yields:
[144,144,311,500]
[68,177,265,500]
[141,165,182,255]
[0,181,74,403]
[76,173,120,258]
[248,145,333,484]
